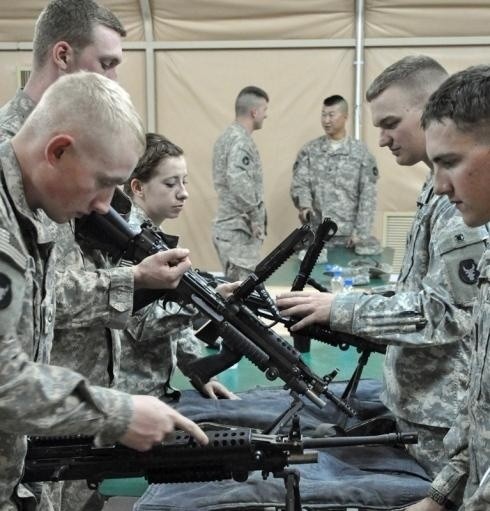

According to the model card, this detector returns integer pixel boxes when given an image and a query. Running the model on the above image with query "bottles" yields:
[330,272,344,293]
[342,278,355,293]
[325,263,345,272]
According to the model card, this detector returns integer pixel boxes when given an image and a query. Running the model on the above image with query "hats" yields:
[347,258,395,280]
[354,236,385,257]
[299,248,329,265]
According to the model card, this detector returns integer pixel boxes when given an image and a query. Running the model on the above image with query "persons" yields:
[290,93,379,247]
[115,131,244,403]
[1,69,212,511]
[404,62,489,509]
[1,1,198,511]
[277,55,489,510]
[210,85,270,281]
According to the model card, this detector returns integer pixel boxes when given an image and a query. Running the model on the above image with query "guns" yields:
[194,218,387,430]
[20,415,418,510]
[75,205,358,420]
